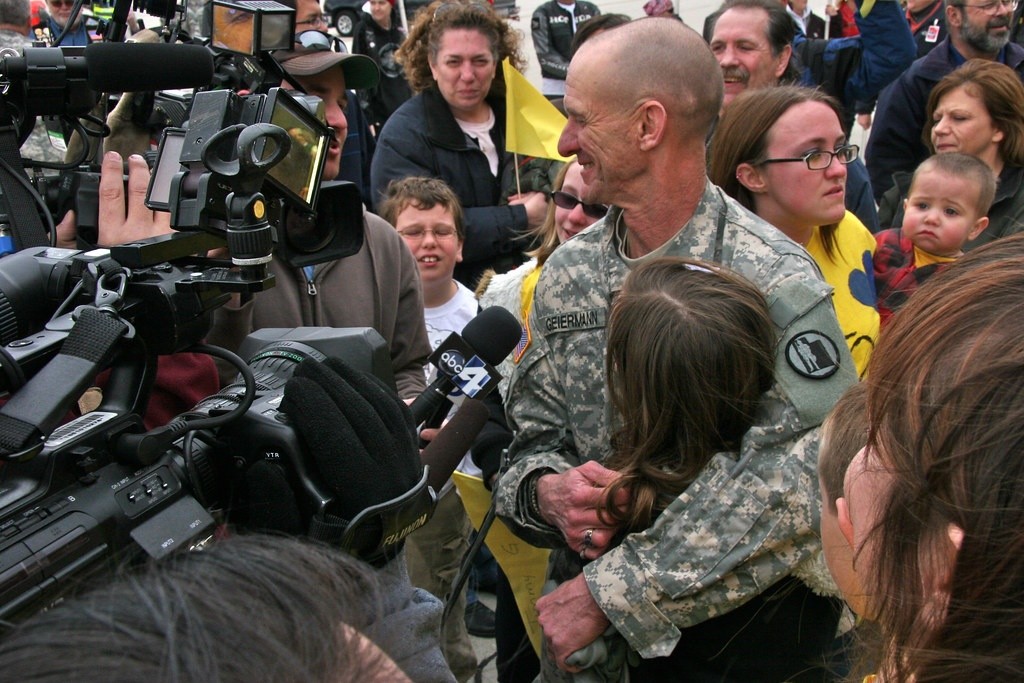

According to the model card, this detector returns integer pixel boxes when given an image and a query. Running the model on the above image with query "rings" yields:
[584,529,593,545]
[579,547,586,560]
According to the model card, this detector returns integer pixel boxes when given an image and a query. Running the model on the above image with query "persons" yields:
[0,1,1024,683]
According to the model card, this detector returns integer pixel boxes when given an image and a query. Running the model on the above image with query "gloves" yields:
[242,355,440,568]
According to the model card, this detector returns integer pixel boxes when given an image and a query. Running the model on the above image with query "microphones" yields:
[65,42,214,93]
[419,399,490,494]
[408,306,523,430]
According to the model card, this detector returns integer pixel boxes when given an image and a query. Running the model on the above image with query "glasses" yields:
[396,222,459,241]
[268,29,348,54]
[748,144,860,171]
[49,0,74,8]
[432,2,490,23]
[952,0,1019,15]
[549,190,609,221]
[295,13,328,29]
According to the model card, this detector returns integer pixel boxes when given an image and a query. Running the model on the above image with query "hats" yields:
[268,37,380,92]
